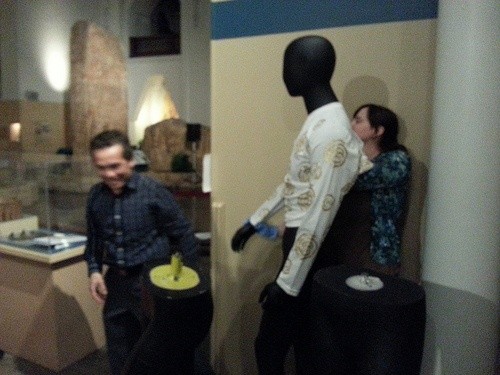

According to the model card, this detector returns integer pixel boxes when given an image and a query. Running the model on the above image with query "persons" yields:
[245,102,414,374]
[230,34,373,375]
[82,128,203,375]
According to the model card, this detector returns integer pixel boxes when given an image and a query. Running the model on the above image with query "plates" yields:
[347,273,382,292]
